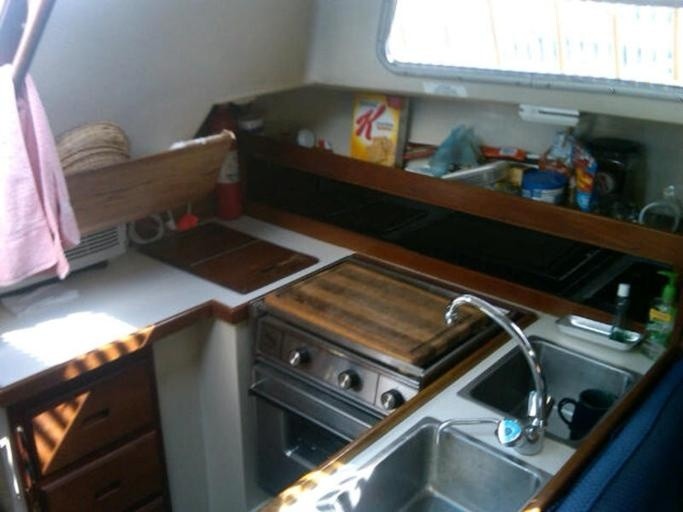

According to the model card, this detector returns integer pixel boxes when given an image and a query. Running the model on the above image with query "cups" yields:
[557,389,619,440]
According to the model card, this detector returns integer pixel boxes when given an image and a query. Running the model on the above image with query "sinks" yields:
[457,336,644,451]
[279,414,553,508]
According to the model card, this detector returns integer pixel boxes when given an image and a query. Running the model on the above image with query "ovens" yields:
[248,367,380,495]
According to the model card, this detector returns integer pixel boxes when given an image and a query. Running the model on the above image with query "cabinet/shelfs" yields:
[0,343,171,512]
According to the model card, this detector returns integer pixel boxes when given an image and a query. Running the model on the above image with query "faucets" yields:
[445,293,553,453]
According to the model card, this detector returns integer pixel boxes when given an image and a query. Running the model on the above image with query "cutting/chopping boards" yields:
[263,260,485,365]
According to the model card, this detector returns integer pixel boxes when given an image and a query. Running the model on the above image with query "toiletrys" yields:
[635,269,679,363]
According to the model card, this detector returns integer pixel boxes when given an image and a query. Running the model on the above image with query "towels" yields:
[1,65,79,291]
